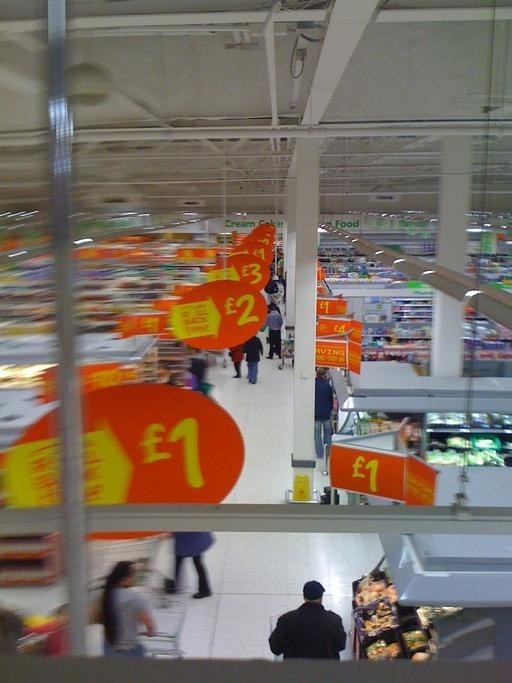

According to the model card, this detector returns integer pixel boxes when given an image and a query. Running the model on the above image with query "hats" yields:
[304,580,324,600]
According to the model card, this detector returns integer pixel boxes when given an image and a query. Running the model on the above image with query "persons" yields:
[315,367,334,459]
[89,560,156,656]
[164,532,218,599]
[185,348,209,390]
[267,580,348,661]
[228,272,287,384]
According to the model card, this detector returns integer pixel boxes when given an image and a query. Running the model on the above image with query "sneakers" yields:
[266,356,281,359]
[234,375,255,384]
[194,592,208,598]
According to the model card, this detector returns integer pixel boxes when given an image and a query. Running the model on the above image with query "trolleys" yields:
[132,595,189,660]
[278,336,295,370]
